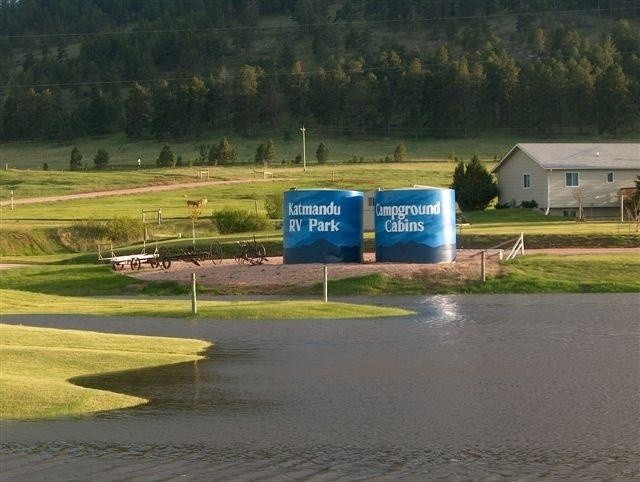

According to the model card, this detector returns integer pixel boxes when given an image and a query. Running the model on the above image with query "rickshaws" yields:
[96,235,222,272]
